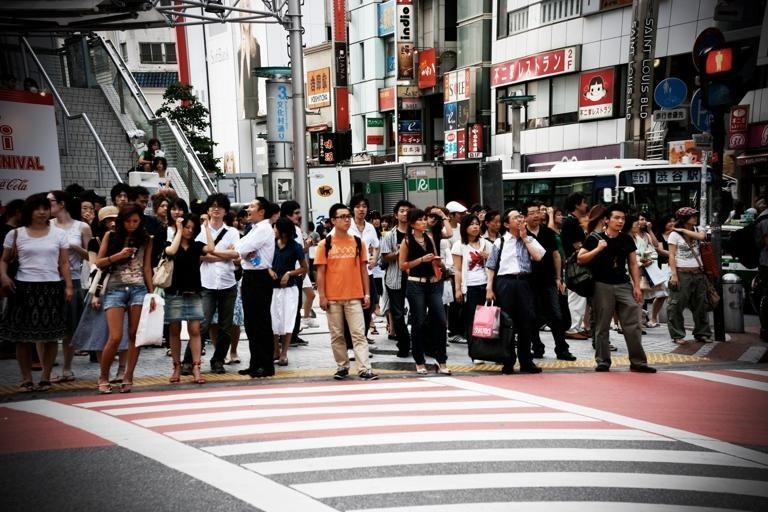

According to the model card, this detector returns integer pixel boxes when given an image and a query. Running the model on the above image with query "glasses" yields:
[334,214,352,219]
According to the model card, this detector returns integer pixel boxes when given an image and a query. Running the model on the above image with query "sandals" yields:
[119,376,133,393]
[98,376,113,392]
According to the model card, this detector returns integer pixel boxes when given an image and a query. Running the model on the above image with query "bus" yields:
[496,154,739,242]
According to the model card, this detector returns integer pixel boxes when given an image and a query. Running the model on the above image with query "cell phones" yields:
[127,238,134,247]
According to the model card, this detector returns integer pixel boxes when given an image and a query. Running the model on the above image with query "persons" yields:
[2,184,319,394]
[127,138,161,177]
[0,74,18,90]
[310,191,767,380]
[151,157,171,189]
[24,78,40,92]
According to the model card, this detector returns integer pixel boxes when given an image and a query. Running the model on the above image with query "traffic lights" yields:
[698,35,744,118]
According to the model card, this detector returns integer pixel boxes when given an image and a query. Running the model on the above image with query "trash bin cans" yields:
[723,273,744,334]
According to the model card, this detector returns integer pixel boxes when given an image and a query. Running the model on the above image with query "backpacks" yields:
[564,232,603,298]
[726,214,768,269]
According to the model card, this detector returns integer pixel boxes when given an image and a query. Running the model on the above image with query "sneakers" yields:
[360,370,378,379]
[645,322,660,328]
[447,335,468,343]
[345,316,413,362]
[334,366,348,380]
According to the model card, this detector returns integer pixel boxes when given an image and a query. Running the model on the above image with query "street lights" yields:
[497,89,537,170]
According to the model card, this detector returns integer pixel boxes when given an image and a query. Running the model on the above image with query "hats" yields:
[97,205,121,223]
[446,200,469,213]
[674,206,699,218]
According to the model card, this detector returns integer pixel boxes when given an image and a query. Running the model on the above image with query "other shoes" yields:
[18,371,75,392]
[237,337,308,380]
[632,365,657,373]
[696,335,712,343]
[167,338,240,381]
[501,351,577,373]
[609,344,618,352]
[595,364,610,372]
[674,338,686,344]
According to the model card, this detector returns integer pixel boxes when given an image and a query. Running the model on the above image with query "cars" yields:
[702,223,757,317]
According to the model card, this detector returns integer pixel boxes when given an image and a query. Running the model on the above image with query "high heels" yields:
[414,362,428,374]
[434,358,452,374]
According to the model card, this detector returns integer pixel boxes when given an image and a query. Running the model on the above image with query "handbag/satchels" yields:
[467,306,514,362]
[700,241,722,282]
[472,299,502,340]
[703,274,721,312]
[87,266,111,298]
[151,257,174,289]
[134,292,166,348]
[639,262,667,292]
